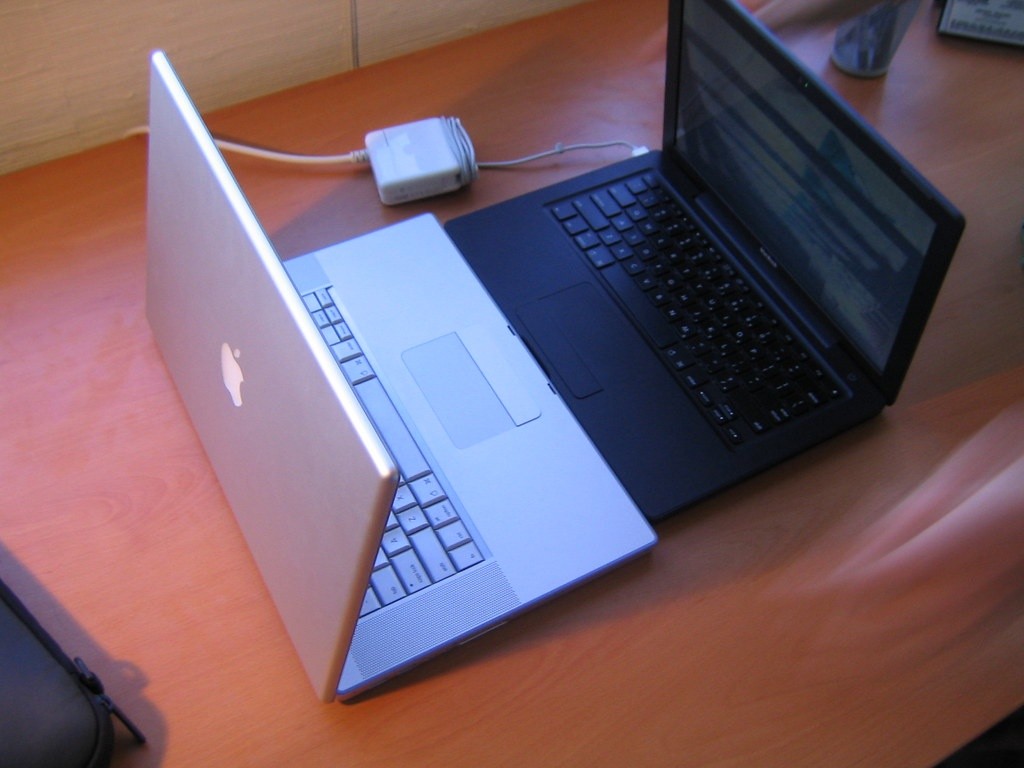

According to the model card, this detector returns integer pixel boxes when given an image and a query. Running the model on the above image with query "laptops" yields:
[445,0,966,528]
[145,51,660,704]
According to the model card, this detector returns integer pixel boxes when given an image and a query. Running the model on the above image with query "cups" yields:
[827,0,923,77]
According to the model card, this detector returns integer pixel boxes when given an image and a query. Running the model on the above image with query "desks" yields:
[0,0,1024,768]
